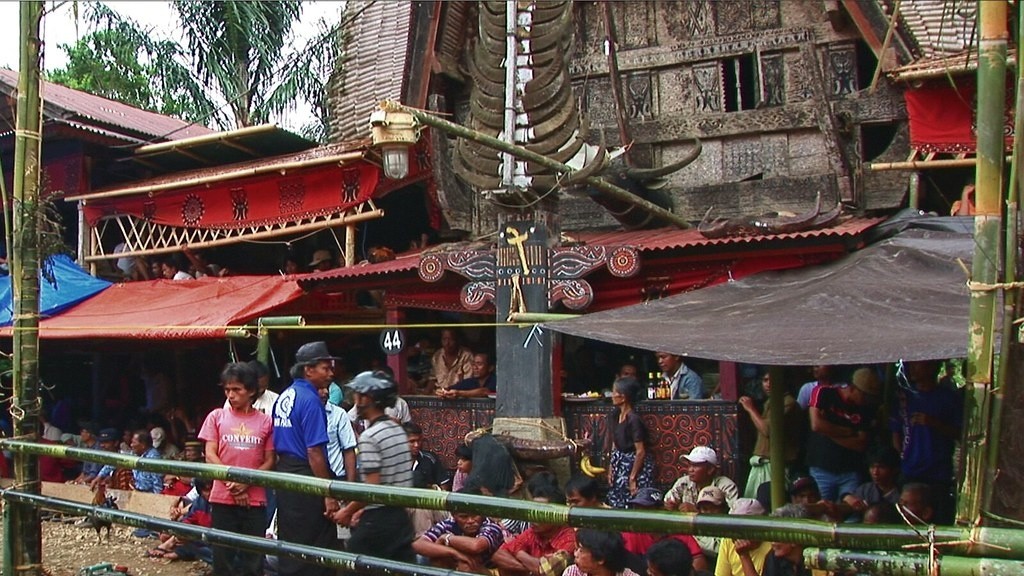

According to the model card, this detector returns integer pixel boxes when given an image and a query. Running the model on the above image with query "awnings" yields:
[538,207,1004,365]
[1,274,307,339]
[0,253,113,326]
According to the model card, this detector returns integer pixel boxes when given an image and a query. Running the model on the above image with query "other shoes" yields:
[134,529,158,536]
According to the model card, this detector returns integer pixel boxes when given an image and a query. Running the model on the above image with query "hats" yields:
[696,485,724,506]
[727,498,765,516]
[620,488,665,507]
[852,368,882,393]
[182,437,201,445]
[151,427,166,448]
[96,428,122,442]
[679,446,719,466]
[784,474,819,496]
[295,341,342,364]
[864,443,902,467]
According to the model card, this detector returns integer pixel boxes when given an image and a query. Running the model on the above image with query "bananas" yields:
[581,455,606,478]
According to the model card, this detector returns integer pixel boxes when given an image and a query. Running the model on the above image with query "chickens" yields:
[91,483,119,545]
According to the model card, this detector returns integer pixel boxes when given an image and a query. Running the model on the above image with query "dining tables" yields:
[401,396,737,499]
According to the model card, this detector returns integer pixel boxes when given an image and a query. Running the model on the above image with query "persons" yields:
[0,219,966,576]
[950,181,975,216]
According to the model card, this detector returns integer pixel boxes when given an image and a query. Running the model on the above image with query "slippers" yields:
[148,547,172,558]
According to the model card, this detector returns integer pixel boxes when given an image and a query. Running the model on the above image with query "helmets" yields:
[343,370,398,408]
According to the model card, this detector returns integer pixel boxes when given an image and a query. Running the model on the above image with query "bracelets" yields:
[445,532,454,546]
[176,476,180,480]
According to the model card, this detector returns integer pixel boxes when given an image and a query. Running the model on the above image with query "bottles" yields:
[647,372,670,399]
